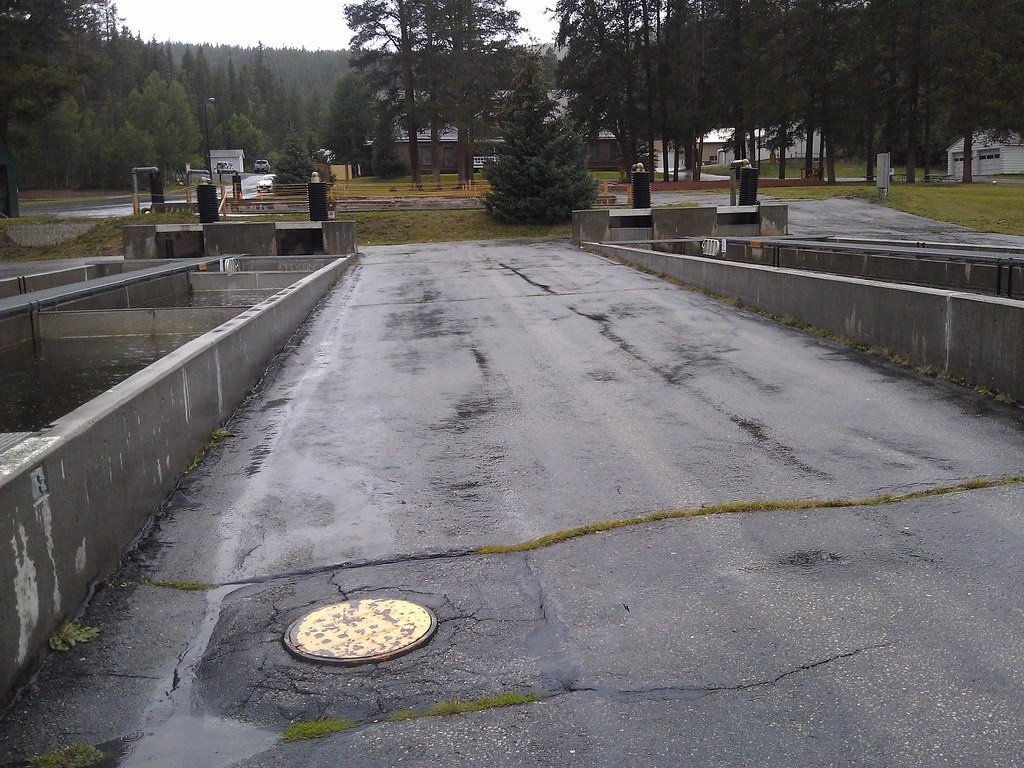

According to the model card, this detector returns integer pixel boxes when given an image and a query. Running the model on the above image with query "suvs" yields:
[217,161,234,174]
[254,159,271,174]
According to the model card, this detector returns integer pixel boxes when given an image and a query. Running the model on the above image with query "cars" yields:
[257,173,277,193]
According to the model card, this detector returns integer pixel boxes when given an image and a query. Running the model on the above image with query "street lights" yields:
[204,97,214,180]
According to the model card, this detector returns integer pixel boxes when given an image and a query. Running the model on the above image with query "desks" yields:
[926,174,954,184]
[892,173,907,182]
[864,176,876,184]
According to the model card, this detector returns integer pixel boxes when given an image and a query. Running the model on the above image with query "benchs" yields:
[893,178,907,182]
[923,177,960,183]
[865,180,876,184]
